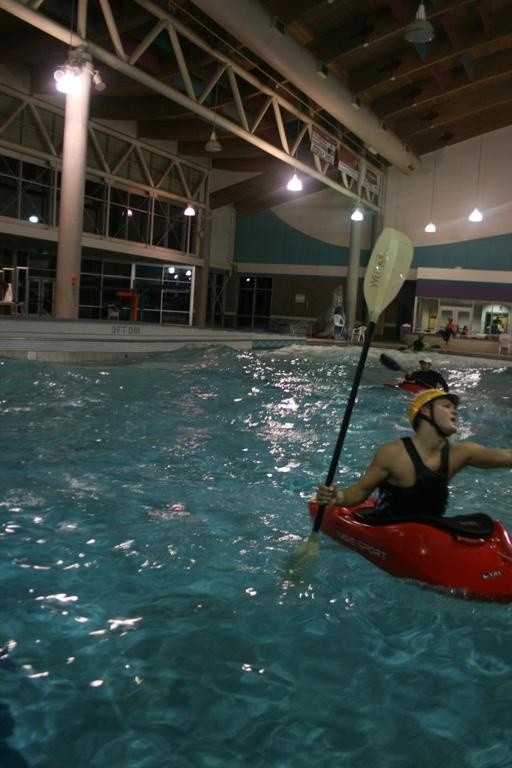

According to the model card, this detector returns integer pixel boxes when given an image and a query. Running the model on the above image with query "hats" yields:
[419,358,432,366]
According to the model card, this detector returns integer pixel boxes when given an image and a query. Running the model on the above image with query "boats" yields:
[307,491,512,607]
[382,381,444,394]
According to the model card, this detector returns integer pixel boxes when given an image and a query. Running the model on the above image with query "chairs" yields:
[350,326,367,342]
[497,333,511,355]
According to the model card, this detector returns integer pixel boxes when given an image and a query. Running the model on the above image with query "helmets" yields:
[407,390,459,431]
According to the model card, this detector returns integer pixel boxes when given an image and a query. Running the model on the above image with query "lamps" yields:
[423,119,483,234]
[204,50,224,154]
[48,46,107,99]
[285,102,365,223]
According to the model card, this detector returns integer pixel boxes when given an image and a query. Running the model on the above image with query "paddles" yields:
[296,228,414,555]
[380,354,434,389]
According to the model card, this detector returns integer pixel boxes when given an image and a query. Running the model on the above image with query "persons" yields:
[445,316,468,335]
[486,316,505,341]
[405,357,449,392]
[413,334,424,351]
[315,389,512,525]
